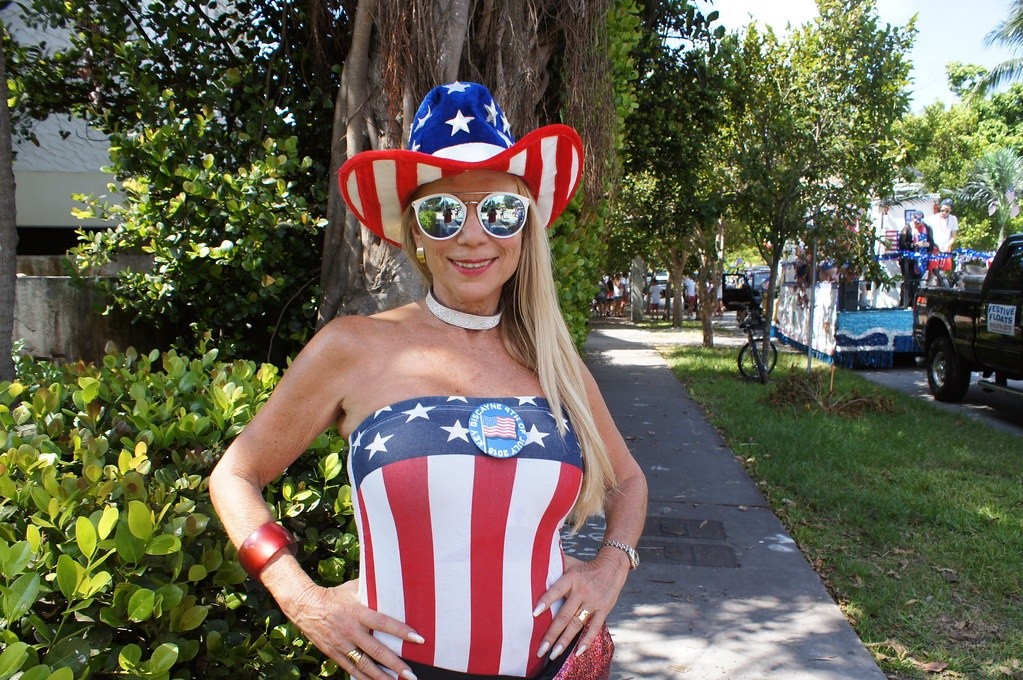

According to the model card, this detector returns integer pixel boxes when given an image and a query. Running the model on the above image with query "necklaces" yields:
[424,284,507,331]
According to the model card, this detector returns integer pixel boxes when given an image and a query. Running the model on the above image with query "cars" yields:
[721,266,780,319]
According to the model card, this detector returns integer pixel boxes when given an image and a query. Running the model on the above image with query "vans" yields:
[643,268,669,299]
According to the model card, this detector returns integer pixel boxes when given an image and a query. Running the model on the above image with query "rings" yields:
[344,647,365,665]
[573,607,593,626]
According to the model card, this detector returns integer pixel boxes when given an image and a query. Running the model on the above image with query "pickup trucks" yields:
[913,234,1023,405]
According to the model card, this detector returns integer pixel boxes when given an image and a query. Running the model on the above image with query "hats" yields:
[336,81,585,246]
[941,198,954,209]
[912,210,924,220]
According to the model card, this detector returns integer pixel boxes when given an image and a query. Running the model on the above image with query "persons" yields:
[592,271,628,318]
[207,81,649,680]
[648,277,663,320]
[682,274,727,321]
[927,199,959,286]
[899,210,933,309]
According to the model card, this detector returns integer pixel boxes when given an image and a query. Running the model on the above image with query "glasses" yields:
[411,192,533,240]
[941,210,951,213]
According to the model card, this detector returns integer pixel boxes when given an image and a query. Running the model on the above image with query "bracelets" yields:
[237,521,299,585]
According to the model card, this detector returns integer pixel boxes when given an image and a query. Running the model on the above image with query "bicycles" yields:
[737,302,778,386]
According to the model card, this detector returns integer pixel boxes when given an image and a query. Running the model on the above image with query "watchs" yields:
[597,539,640,572]
